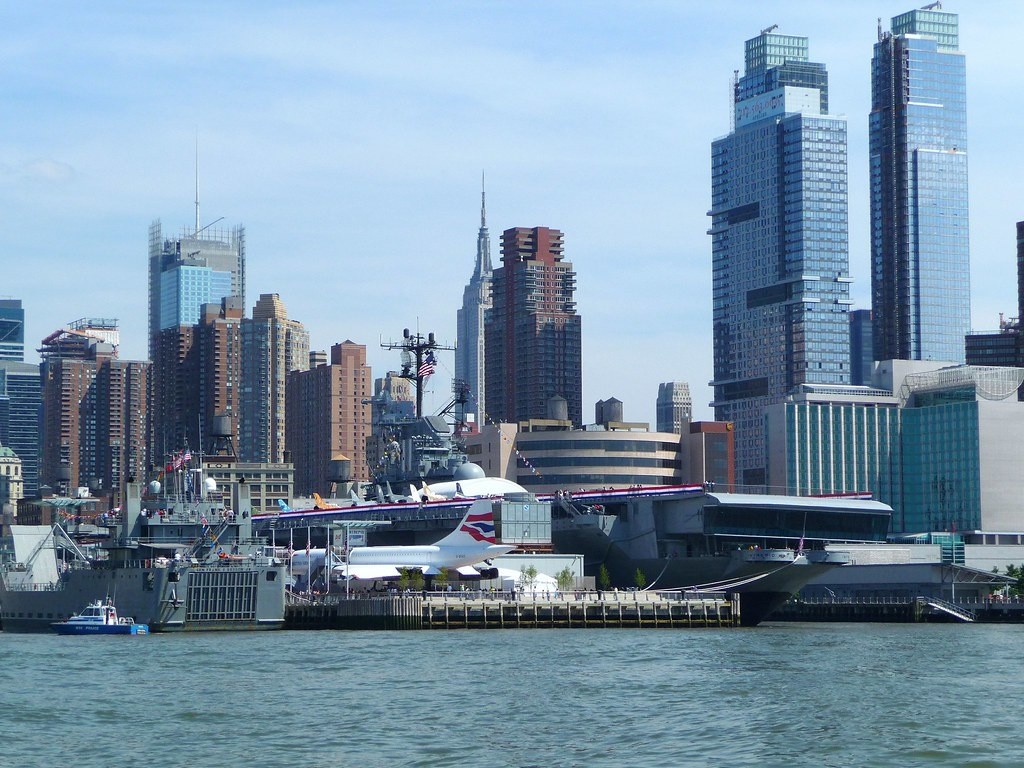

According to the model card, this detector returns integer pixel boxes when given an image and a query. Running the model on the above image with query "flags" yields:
[288,540,295,555]
[344,530,347,552]
[184,470,229,559]
[325,541,330,557]
[418,355,435,377]
[460,381,539,477]
[305,542,311,557]
[173,448,191,470]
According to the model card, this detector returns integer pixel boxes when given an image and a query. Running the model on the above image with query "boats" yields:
[532,480,895,622]
[48,595,149,635]
[0,412,288,631]
[252,316,554,593]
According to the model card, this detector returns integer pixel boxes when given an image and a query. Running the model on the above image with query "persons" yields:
[103,511,109,523]
[701,480,716,494]
[49,581,53,591]
[146,509,167,523]
[107,595,112,606]
[219,508,237,523]
[555,484,643,498]
[737,544,762,550]
[69,563,73,573]
[987,592,1019,603]
[342,583,618,601]
